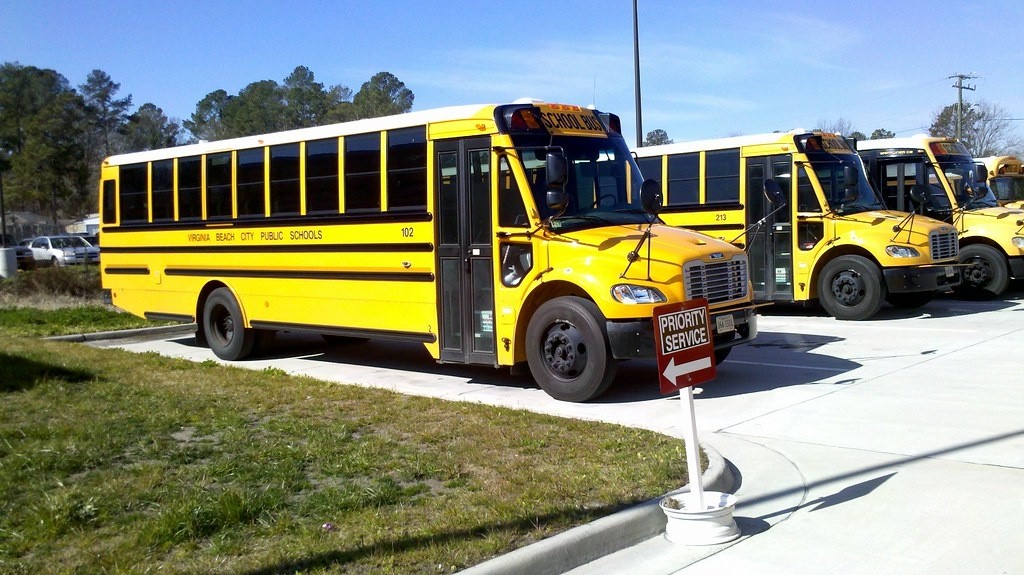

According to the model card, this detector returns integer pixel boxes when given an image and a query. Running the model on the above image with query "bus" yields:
[884,154,1024,212]
[83,218,100,245]
[99,99,787,404]
[442,130,989,320]
[775,133,1024,300]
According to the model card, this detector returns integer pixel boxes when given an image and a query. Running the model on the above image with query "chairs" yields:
[121,187,358,221]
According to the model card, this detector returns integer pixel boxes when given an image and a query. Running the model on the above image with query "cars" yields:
[0,234,34,269]
[27,235,98,269]
[20,237,33,248]
